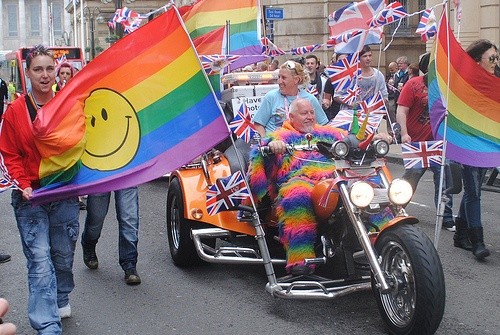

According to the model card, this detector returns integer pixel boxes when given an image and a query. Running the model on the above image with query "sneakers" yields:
[125,267,140,284]
[82,243,98,269]
[59,303,71,318]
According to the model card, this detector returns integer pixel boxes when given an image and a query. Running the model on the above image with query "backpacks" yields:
[317,72,341,120]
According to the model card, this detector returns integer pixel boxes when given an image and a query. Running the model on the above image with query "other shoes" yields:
[237,210,244,219]
[292,263,310,274]
[79,200,87,210]
[0,253,11,262]
[442,221,456,231]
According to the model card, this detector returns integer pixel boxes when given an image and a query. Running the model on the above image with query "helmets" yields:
[311,179,341,221]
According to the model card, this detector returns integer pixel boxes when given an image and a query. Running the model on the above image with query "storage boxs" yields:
[221,84,279,118]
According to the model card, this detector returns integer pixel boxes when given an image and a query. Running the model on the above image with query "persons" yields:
[80,185,142,284]
[388,57,420,122]
[453,39,500,258]
[334,46,388,110]
[397,53,456,231]
[252,59,329,138]
[322,54,349,119]
[0,45,87,335]
[305,55,326,108]
[249,98,392,276]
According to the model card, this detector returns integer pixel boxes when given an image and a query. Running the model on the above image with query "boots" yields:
[469,226,490,259]
[453,216,473,250]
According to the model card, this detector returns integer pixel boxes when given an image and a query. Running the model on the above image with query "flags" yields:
[205,170,250,217]
[262,0,438,56]
[179,0,262,99]
[428,12,500,170]
[28,7,230,199]
[307,51,387,135]
[107,7,142,32]
[230,102,256,143]
[401,141,446,169]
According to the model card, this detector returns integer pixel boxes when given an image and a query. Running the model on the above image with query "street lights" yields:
[78,6,106,60]
[60,30,74,46]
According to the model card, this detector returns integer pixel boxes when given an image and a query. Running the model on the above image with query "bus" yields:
[4,46,85,103]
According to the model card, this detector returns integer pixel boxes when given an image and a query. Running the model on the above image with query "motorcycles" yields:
[166,73,451,335]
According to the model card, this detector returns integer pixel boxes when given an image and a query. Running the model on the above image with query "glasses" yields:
[284,61,299,76]
[480,55,499,63]
